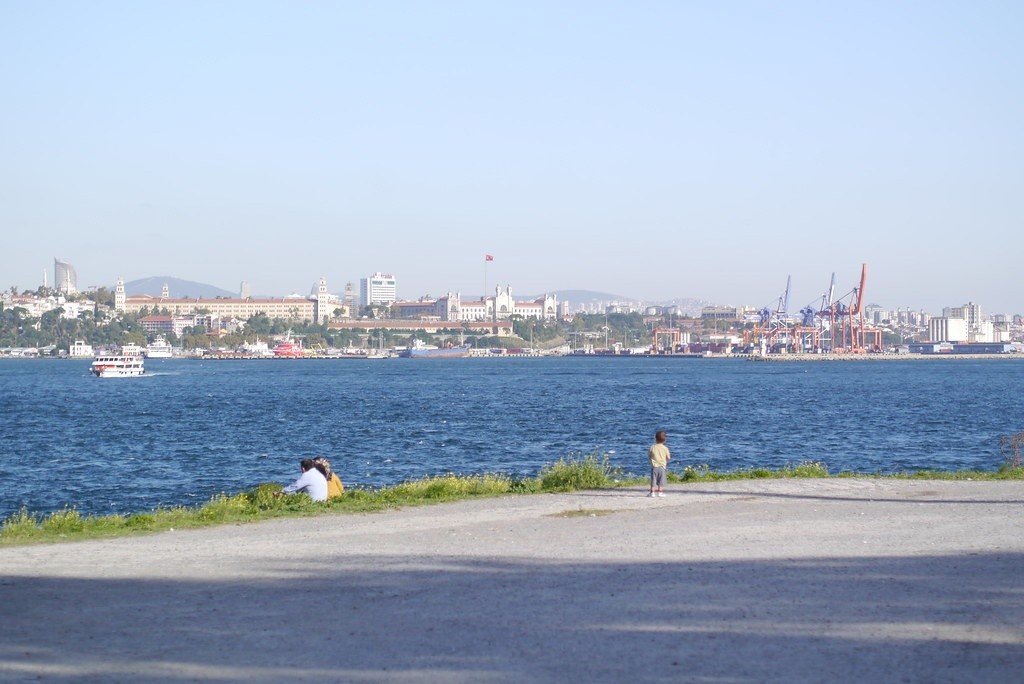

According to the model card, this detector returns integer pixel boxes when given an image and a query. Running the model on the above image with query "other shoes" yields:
[647,493,655,497]
[657,492,665,496]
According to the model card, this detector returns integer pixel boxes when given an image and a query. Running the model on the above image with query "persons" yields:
[645,432,671,497]
[273,458,328,503]
[313,457,345,500]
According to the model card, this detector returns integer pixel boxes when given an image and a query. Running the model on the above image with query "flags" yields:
[486,255,493,261]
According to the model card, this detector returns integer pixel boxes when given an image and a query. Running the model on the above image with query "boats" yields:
[272,327,304,356]
[236,333,269,354]
[143,333,172,359]
[89,353,145,378]
[57,353,72,360]
[399,334,471,359]
